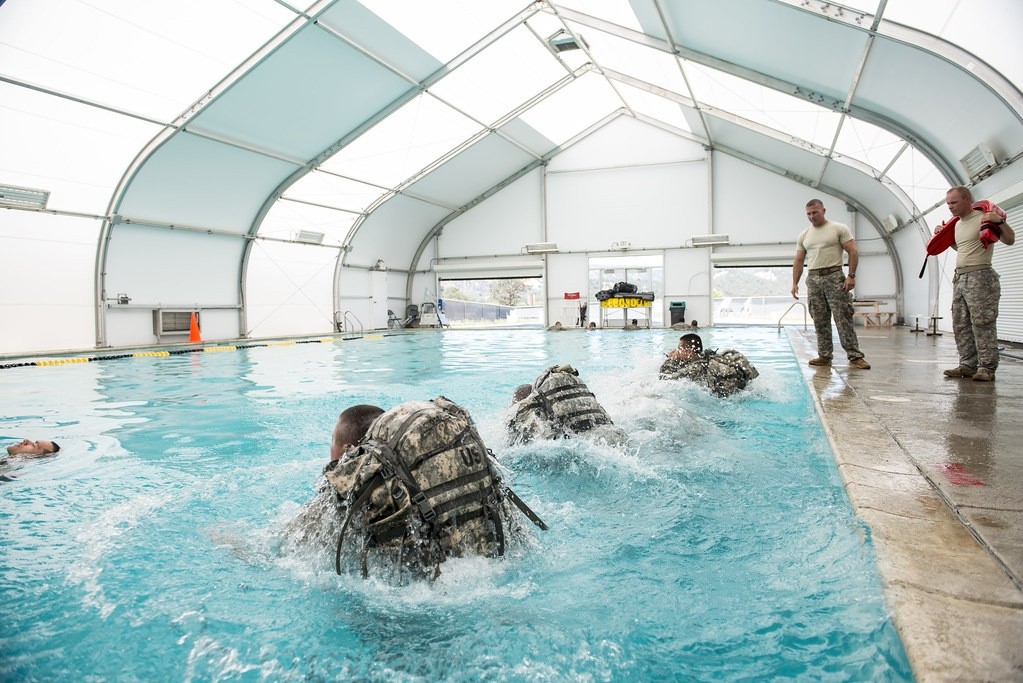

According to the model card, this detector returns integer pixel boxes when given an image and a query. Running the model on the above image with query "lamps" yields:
[881,213,898,232]
[525,240,559,254]
[371,259,390,272]
[958,142,997,180]
[0,184,51,210]
[293,230,325,244]
[691,233,730,247]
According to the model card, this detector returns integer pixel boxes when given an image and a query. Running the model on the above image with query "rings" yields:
[852,286,853,288]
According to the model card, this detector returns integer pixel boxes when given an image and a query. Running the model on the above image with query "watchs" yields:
[848,273,855,278]
[996,217,1006,225]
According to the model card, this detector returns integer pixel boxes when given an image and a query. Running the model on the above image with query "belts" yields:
[955,265,991,274]
[809,267,841,275]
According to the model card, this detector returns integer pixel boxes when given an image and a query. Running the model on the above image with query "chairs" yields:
[558,291,583,325]
[387,309,403,328]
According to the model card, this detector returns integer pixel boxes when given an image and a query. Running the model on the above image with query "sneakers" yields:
[943,364,978,377]
[849,357,871,369]
[808,357,832,367]
[973,369,995,380]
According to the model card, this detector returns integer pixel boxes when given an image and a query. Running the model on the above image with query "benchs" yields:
[855,310,901,327]
[909,314,943,336]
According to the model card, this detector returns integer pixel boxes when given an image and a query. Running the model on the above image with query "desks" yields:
[599,291,655,328]
[851,300,888,326]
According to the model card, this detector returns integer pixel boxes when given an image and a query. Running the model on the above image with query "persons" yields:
[548,322,562,331]
[588,323,596,330]
[503,385,630,449]
[7,439,59,459]
[935,185,1015,381]
[659,334,738,398]
[687,320,700,331]
[293,405,386,587]
[791,200,870,369]
[624,319,640,330]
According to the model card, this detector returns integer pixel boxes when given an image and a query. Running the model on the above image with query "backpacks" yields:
[510,367,612,436]
[683,348,758,398]
[321,393,550,587]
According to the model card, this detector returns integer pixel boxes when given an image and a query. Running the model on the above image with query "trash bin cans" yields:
[669,302,686,326]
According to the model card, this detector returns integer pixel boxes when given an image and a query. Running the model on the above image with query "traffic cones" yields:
[189,311,200,342]
